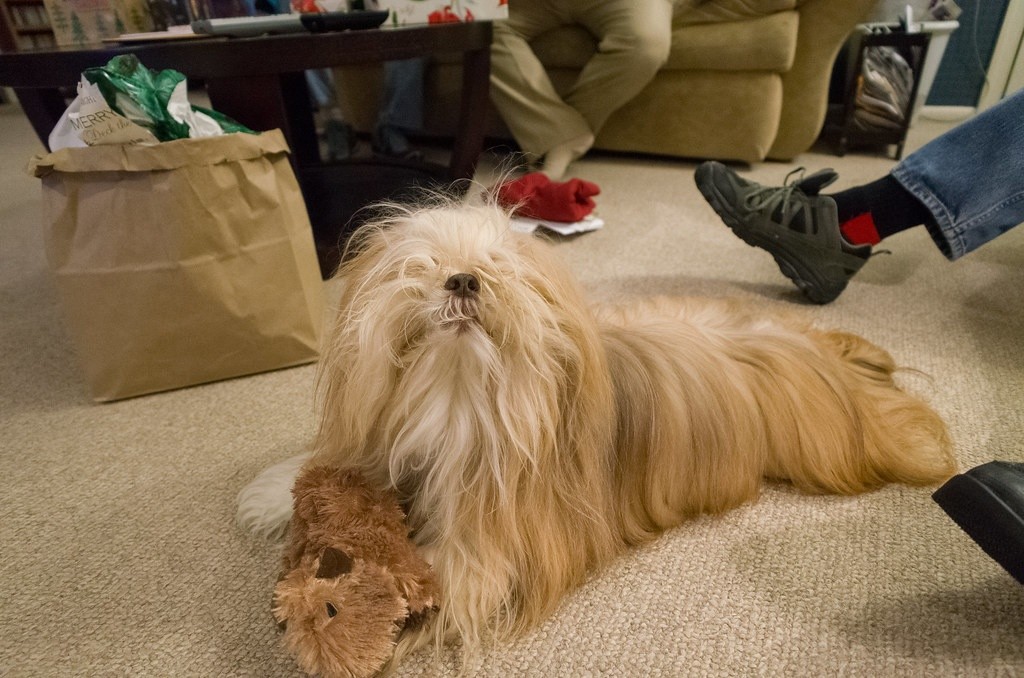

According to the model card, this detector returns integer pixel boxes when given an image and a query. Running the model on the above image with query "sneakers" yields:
[695,160,892,305]
[931,457,1024,586]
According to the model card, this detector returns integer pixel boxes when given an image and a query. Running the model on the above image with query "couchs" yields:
[349,0,877,164]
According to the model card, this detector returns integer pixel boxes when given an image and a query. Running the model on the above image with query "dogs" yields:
[235,153,960,677]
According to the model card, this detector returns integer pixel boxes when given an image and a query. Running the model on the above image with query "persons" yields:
[930,460,1024,587]
[694,91,1024,304]
[490,0,673,181]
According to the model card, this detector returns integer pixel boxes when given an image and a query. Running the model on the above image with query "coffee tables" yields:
[0,20,494,282]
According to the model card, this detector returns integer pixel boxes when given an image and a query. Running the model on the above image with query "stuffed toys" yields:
[270,463,440,678]
